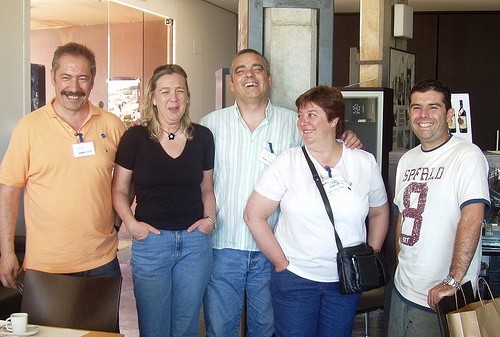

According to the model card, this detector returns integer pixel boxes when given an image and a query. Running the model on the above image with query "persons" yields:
[126,49,364,337]
[386,78,492,337]
[1,41,134,333]
[243,85,390,337]
[115,64,216,336]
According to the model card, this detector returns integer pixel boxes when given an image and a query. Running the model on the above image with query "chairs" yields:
[436,280,474,337]
[21,268,124,333]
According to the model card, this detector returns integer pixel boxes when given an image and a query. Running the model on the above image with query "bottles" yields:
[458,100,467,133]
[449,112,456,133]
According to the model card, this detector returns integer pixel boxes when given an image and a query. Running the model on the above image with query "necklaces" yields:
[160,124,182,141]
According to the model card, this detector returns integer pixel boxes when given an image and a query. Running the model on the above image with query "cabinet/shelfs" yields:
[337,87,395,187]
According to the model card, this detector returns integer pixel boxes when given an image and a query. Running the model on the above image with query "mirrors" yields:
[22,0,174,136]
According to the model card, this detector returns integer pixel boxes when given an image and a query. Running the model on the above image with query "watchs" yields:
[443,275,461,290]
[205,216,216,230]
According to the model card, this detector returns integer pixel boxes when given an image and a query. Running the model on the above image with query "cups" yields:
[5,313,28,333]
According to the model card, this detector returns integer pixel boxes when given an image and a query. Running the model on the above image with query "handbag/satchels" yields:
[335,242,391,296]
[444,276,500,337]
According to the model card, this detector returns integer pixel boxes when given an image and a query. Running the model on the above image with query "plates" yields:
[0,325,39,336]
